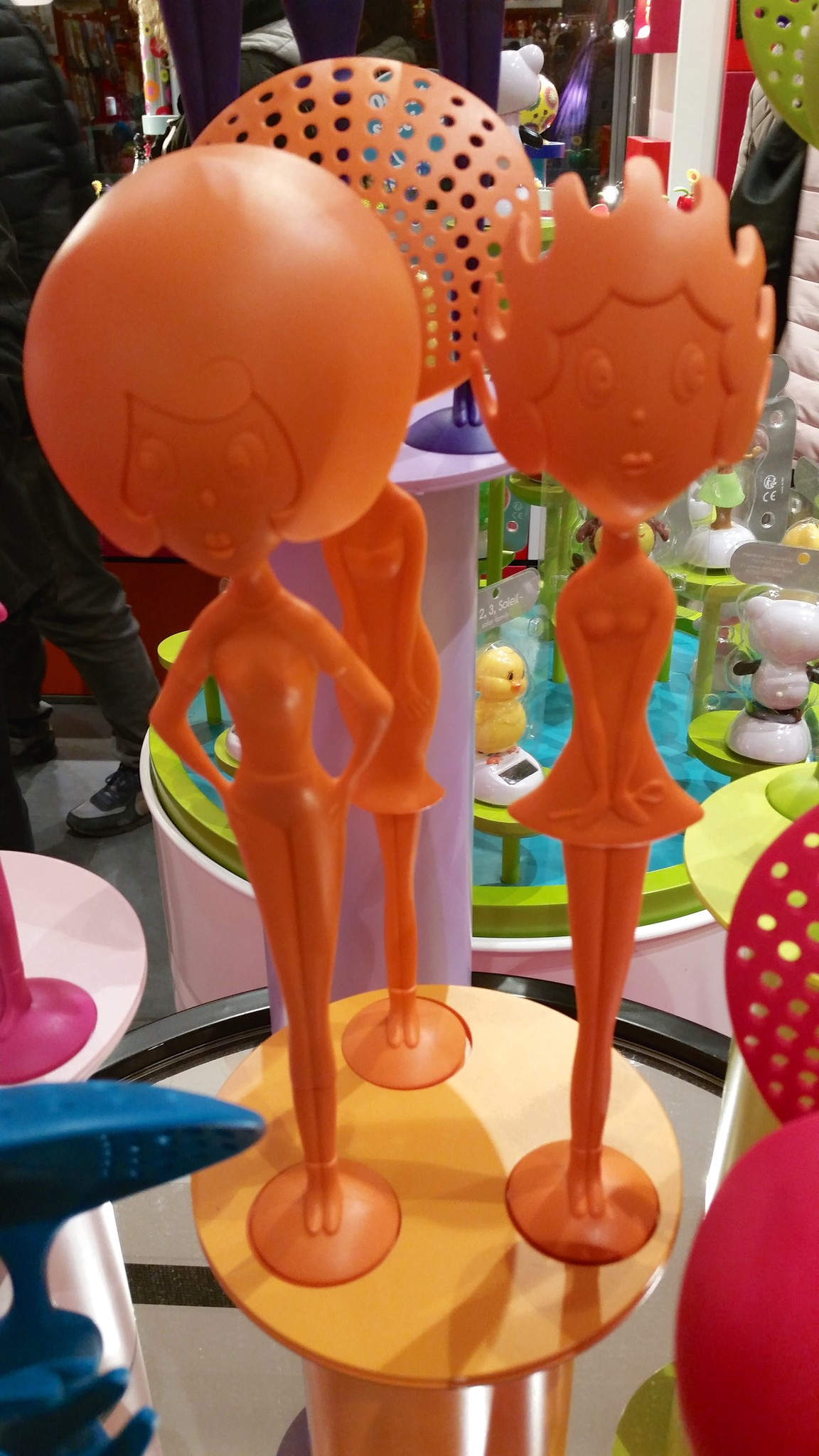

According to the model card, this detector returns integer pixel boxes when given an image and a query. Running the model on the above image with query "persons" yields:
[0,0,163,836]
[25,141,423,1235]
[476,154,774,1217]
[319,479,448,1045]
[431,0,504,425]
[0,855,33,1041]
[731,6,819,485]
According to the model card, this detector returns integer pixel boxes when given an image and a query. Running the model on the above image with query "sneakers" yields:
[6,720,60,763]
[67,763,152,836]
